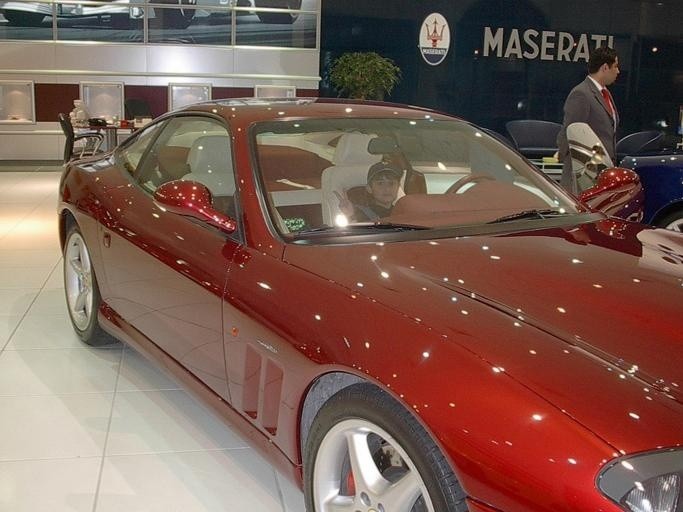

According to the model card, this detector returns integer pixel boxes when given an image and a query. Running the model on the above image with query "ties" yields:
[602,89,612,116]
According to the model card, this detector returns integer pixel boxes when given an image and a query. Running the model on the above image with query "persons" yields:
[556,46,620,194]
[331,160,403,224]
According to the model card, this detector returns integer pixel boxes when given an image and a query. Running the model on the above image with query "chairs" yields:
[58,113,105,166]
[124,98,152,133]
[180,130,407,232]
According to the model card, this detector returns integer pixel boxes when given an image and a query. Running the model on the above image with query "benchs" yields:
[505,120,664,159]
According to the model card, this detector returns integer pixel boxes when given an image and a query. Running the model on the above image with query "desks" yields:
[72,123,141,153]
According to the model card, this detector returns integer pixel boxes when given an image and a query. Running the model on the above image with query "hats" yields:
[367,162,403,185]
